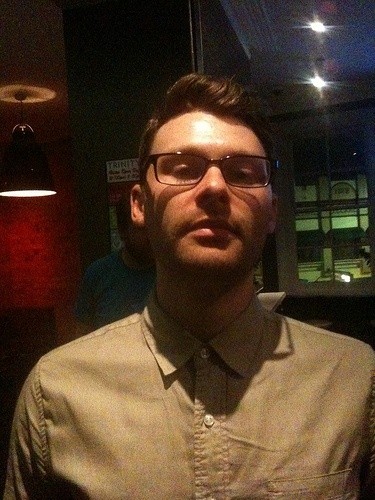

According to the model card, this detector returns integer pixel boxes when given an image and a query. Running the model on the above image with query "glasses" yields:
[137,150,281,187]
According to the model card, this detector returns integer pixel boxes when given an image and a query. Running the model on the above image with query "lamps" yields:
[0,86,56,197]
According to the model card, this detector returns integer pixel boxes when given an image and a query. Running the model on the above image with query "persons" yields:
[3,72,375,500]
